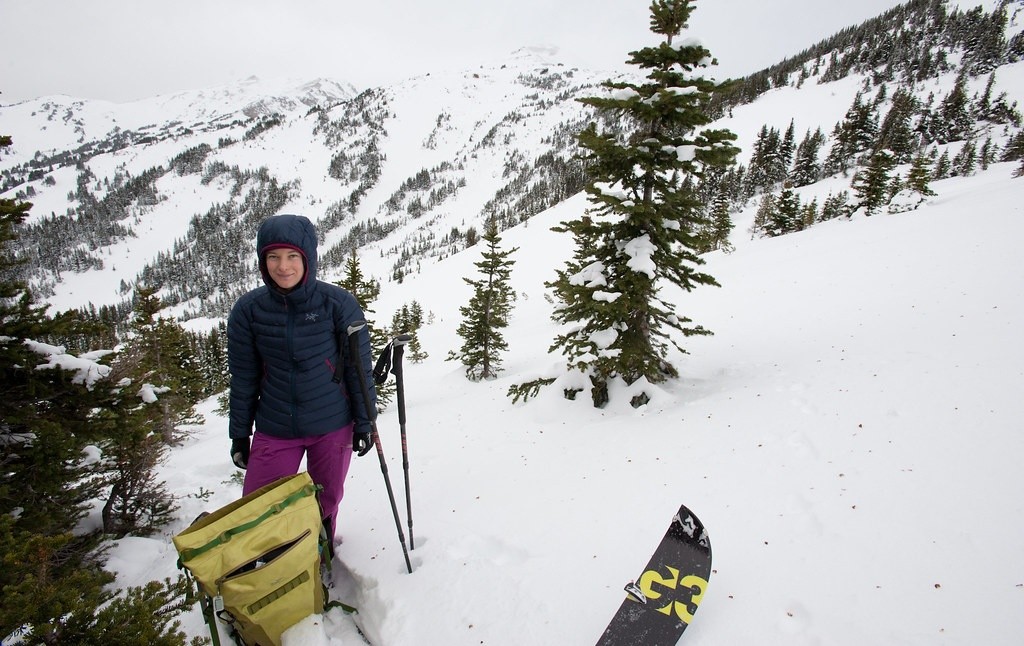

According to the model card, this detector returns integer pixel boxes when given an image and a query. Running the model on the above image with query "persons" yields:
[227,215,378,559]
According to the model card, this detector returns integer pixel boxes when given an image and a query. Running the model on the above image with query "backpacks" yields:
[172,471,358,646]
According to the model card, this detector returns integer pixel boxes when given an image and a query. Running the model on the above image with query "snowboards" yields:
[595,502,716,645]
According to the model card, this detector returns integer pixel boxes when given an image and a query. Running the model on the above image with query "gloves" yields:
[352,420,375,457]
[230,437,250,469]
